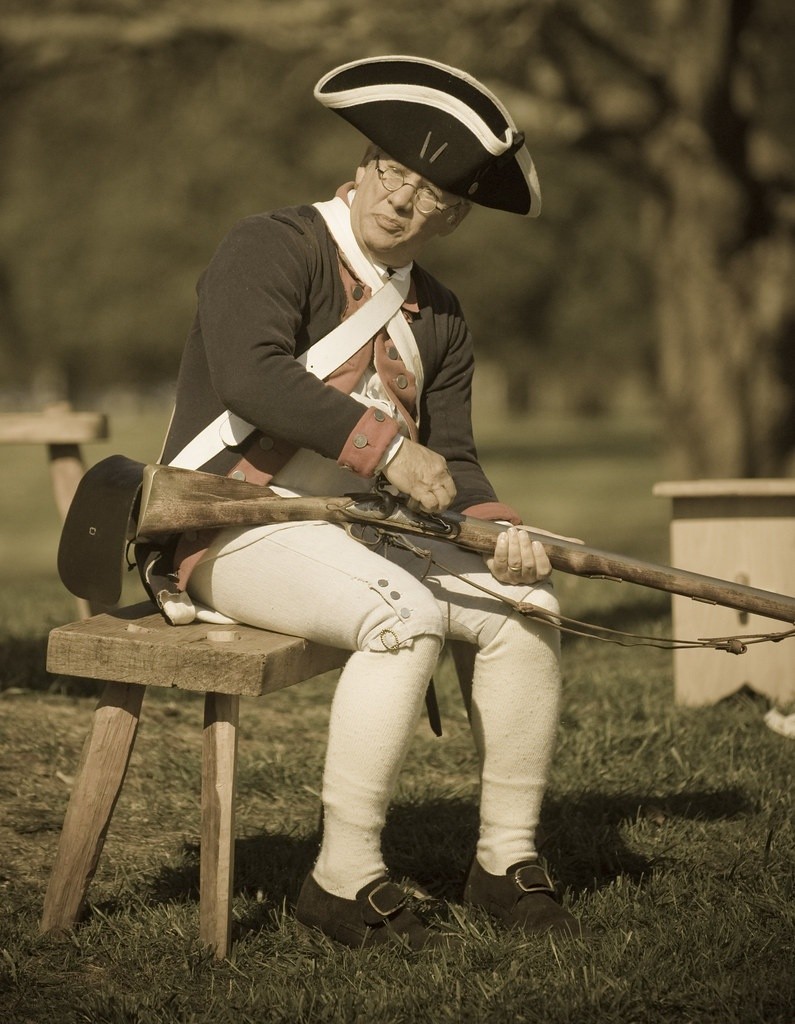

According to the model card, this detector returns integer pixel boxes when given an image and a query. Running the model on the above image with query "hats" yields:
[314,55,541,218]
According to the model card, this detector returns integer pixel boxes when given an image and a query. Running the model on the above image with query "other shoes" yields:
[464,853,586,941]
[293,871,464,965]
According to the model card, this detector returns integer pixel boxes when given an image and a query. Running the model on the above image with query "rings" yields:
[510,567,522,571]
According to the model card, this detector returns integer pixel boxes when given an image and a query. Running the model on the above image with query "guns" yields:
[134,457,795,630]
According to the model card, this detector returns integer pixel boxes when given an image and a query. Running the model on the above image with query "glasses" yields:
[375,155,462,214]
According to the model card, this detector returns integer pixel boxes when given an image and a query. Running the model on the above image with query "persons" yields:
[132,54,590,963]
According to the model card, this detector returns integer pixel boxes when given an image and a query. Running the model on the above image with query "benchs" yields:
[38,596,487,969]
